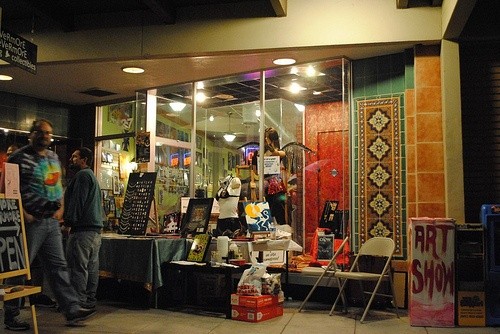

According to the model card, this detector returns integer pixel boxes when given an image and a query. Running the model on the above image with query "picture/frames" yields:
[183,197,215,234]
[112,174,121,195]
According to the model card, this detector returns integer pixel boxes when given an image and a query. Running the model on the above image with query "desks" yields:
[99,236,188,309]
[169,262,283,318]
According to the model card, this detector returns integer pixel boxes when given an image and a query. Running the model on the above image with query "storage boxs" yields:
[230,294,283,323]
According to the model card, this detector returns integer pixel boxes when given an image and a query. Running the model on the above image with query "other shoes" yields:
[4,313,32,330]
[31,295,58,307]
[65,308,96,324]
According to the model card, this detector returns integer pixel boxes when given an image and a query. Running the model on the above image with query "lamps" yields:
[223,111,236,143]
[169,103,186,112]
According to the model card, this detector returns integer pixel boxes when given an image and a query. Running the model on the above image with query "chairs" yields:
[330,237,396,322]
[298,236,350,312]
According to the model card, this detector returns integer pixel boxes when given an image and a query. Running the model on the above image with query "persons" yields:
[252,127,287,224]
[0,119,103,330]
[215,174,241,235]
